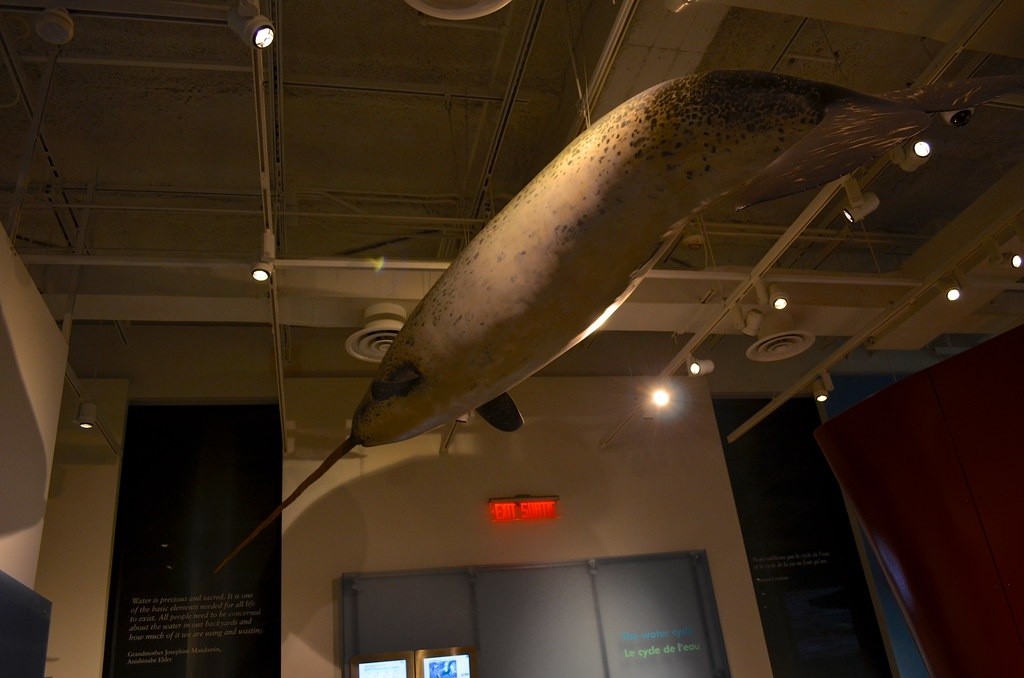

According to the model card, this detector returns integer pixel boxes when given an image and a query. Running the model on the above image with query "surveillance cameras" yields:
[940,106,974,128]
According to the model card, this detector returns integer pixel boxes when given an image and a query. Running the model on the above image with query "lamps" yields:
[71,402,97,429]
[899,139,931,173]
[733,304,764,337]
[686,355,714,377]
[1008,248,1024,272]
[843,178,880,223]
[768,284,792,312]
[811,373,834,403]
[938,275,960,302]
[251,262,273,282]
[227,0,275,50]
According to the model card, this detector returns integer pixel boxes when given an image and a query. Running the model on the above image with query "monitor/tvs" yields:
[349,650,415,678]
[415,645,479,678]
